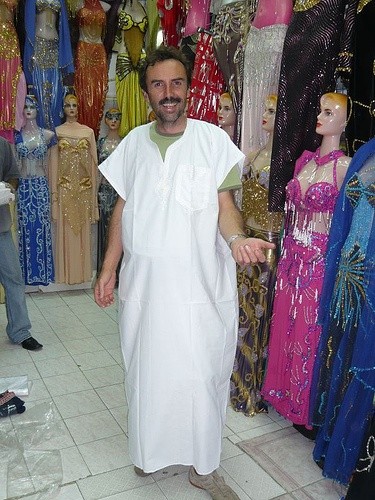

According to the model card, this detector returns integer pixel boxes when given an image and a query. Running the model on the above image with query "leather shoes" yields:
[21,337,43,350]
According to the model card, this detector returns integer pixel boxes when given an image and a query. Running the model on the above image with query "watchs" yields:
[228,234,248,250]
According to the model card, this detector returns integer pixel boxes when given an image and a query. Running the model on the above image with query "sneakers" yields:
[189,465,240,500]
[134,466,152,477]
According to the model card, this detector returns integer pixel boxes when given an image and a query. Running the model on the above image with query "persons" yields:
[0,136,43,350]
[261,93,352,437]
[1,1,375,148]
[230,94,281,416]
[53,94,98,285]
[96,108,124,278]
[95,46,275,500]
[308,136,375,483]
[10,96,59,285]
